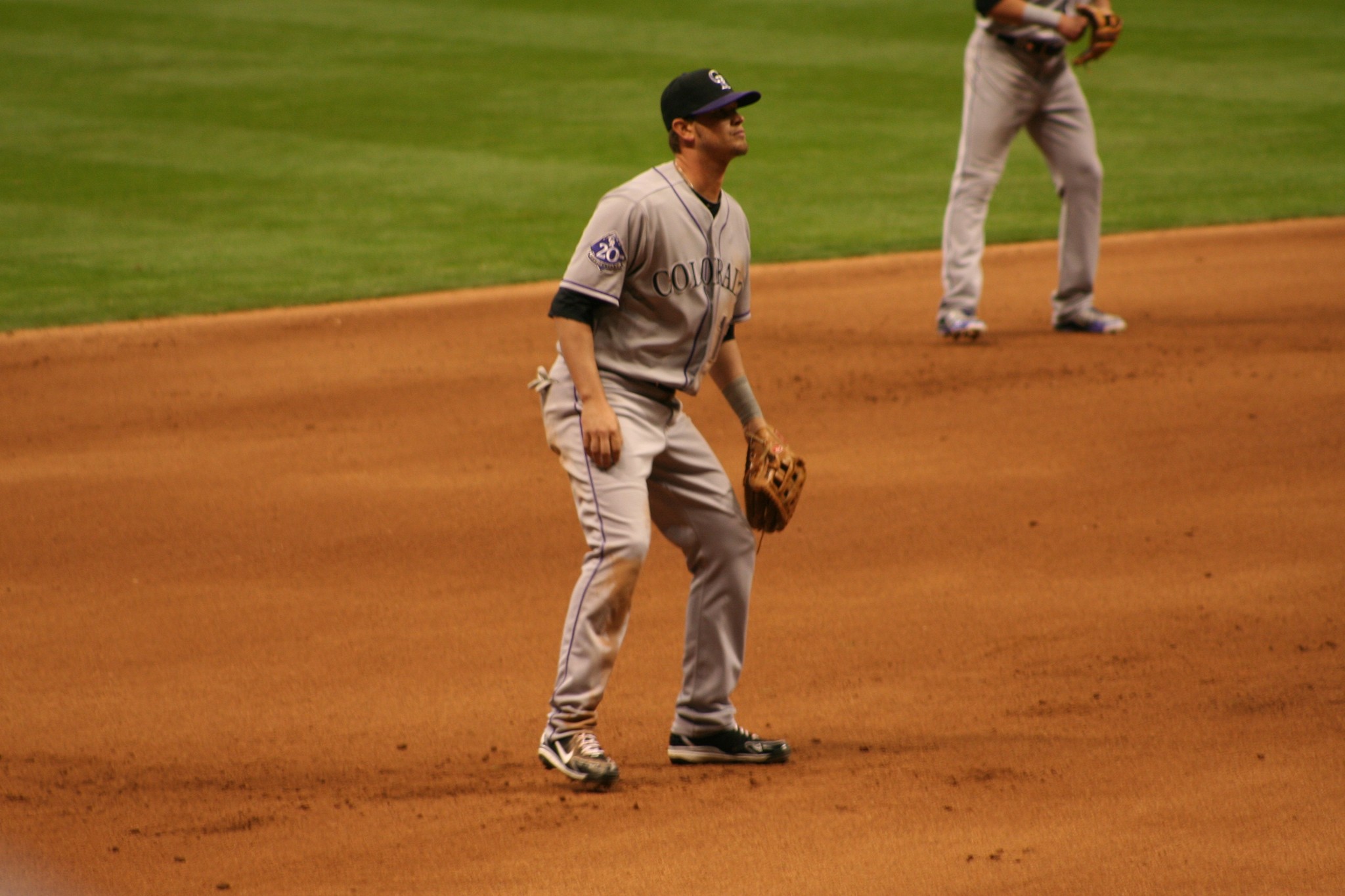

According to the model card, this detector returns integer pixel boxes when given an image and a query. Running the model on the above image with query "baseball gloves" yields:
[743,421,807,531]
[1073,3,1123,65]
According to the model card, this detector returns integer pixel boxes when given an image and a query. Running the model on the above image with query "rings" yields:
[1075,27,1082,32]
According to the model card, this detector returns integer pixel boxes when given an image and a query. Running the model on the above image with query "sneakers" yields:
[537,732,619,782]
[1053,303,1128,335]
[666,720,791,765]
[936,309,986,342]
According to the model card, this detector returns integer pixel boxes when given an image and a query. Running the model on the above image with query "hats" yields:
[660,68,761,132]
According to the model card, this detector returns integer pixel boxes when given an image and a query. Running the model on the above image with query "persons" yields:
[935,0,1127,332]
[529,66,789,782]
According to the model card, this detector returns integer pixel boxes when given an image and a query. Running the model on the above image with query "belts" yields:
[597,366,675,395]
[983,27,1064,56]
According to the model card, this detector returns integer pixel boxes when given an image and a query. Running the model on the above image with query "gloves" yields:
[527,365,552,392]
